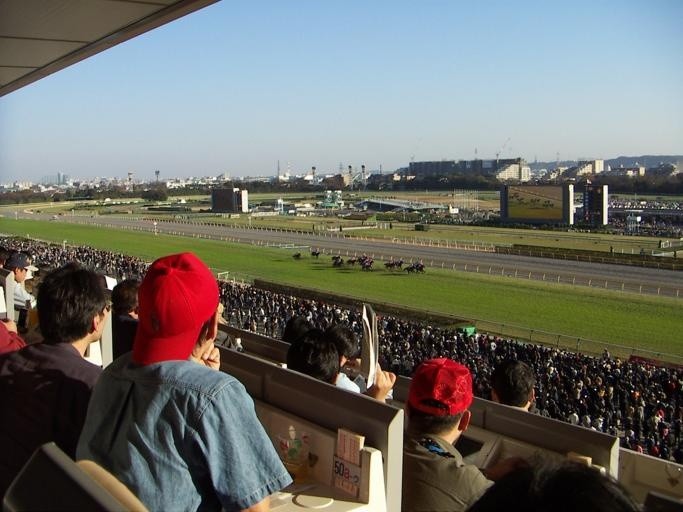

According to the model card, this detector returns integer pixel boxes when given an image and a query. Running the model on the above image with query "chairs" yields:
[75,459,148,512]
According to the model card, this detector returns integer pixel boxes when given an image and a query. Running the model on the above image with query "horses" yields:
[384,260,403,270]
[402,264,427,275]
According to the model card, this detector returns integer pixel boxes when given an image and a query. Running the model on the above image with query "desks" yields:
[251,401,386,512]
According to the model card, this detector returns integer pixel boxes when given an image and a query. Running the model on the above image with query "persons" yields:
[282,294,396,403]
[598,347,682,463]
[74,252,293,511]
[336,253,422,268]
[1,263,105,497]
[401,358,494,510]
[393,319,603,432]
[216,279,286,339]
[490,359,536,412]
[466,458,640,511]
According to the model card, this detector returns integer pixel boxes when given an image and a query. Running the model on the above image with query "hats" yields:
[5,254,39,271]
[132,252,219,366]
[409,357,473,416]
